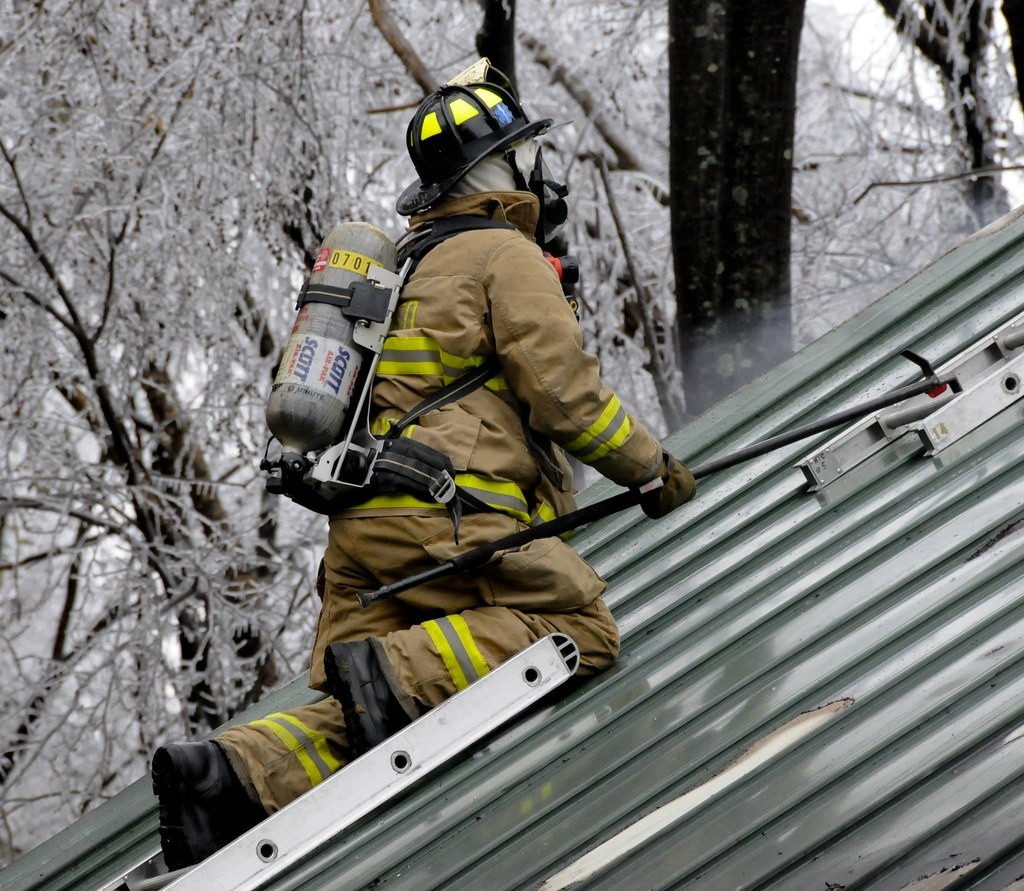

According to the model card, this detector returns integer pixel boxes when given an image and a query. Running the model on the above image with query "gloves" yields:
[641,447,699,520]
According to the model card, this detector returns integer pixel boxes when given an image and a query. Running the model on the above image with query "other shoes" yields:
[151,739,263,873]
[323,639,394,757]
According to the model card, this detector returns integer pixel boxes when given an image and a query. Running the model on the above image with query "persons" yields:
[152,82,696,873]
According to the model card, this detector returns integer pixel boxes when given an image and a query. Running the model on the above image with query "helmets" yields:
[395,81,554,216]
